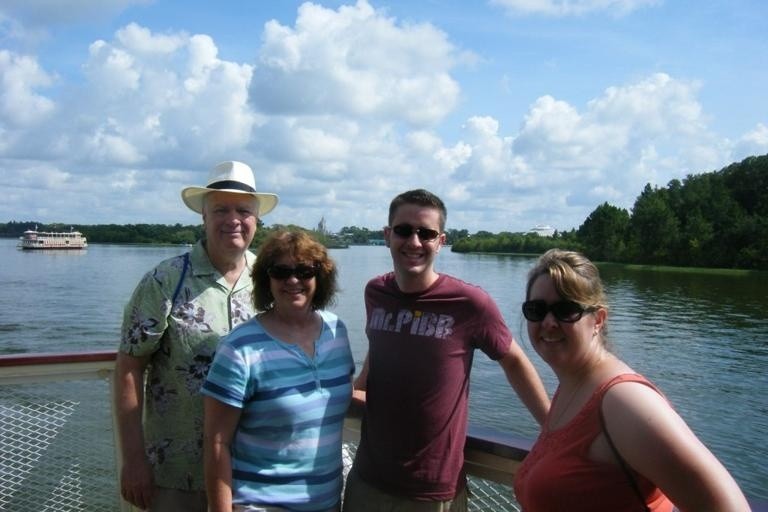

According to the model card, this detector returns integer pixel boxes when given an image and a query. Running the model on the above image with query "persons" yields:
[511,244,754,510]
[199,229,367,511]
[342,190,552,512]
[111,160,282,512]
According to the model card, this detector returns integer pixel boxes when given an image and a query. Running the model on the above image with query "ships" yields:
[15,230,89,249]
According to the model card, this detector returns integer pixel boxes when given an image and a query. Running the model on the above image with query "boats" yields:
[0,350,768,512]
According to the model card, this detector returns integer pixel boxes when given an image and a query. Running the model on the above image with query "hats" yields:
[181,162,278,211]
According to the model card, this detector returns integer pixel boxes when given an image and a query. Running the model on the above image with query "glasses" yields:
[522,296,599,323]
[389,222,441,241]
[270,258,319,281]
